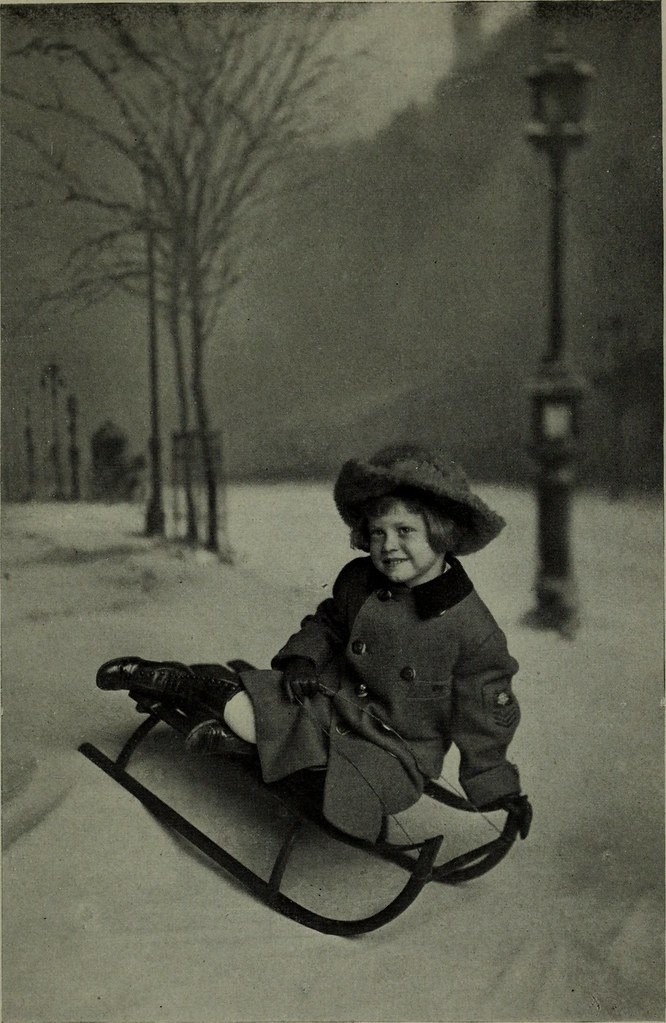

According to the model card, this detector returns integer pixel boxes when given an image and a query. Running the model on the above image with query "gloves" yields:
[282,656,318,706]
[489,792,533,838]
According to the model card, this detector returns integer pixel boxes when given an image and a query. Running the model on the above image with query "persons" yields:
[97,444,532,840]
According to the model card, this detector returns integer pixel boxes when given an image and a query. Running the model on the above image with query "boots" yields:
[95,656,240,721]
[184,719,255,755]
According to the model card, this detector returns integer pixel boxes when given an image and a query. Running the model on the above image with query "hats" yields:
[334,444,507,556]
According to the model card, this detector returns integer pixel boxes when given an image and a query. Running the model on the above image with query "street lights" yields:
[519,28,594,635]
